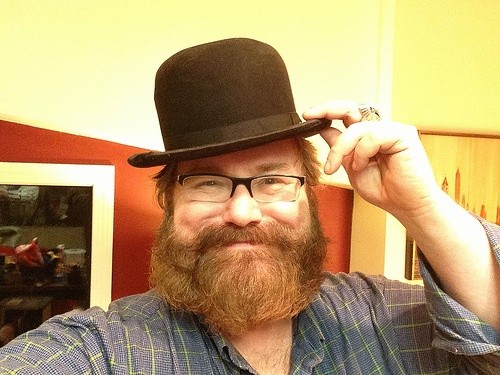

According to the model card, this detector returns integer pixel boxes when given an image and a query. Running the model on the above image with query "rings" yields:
[359,102,382,122]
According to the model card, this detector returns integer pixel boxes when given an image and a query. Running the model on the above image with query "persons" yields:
[0,37,500,375]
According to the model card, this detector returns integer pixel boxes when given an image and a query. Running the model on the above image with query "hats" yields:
[128,36,332,168]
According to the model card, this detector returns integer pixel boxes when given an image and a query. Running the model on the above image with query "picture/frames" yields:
[0,161,116,347]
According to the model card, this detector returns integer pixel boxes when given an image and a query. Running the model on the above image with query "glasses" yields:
[170,171,307,204]
